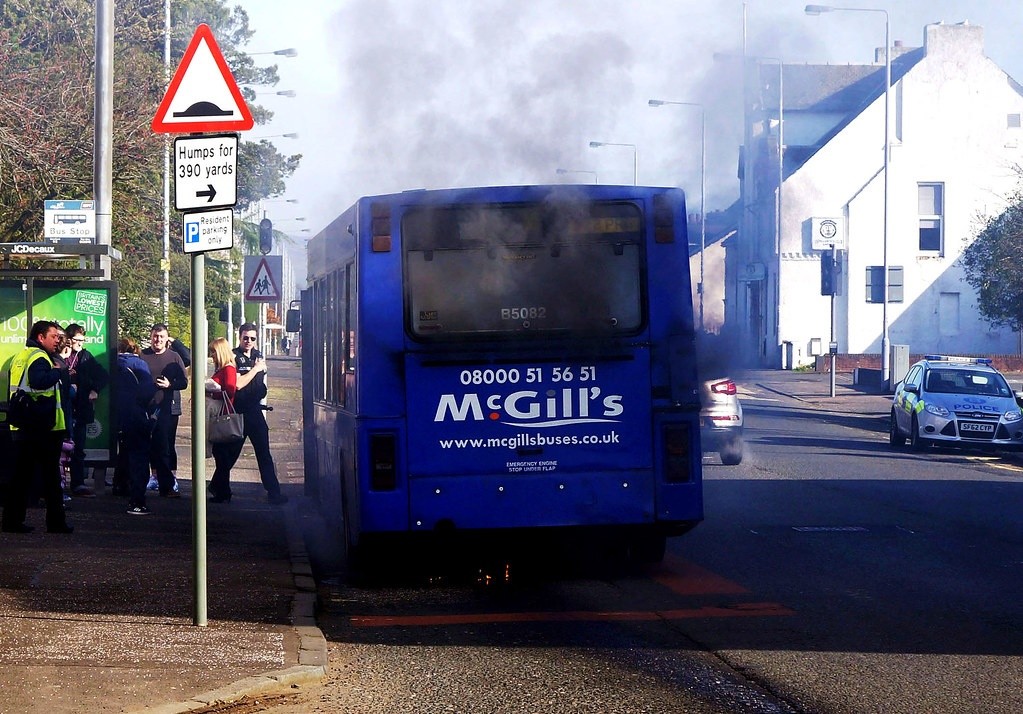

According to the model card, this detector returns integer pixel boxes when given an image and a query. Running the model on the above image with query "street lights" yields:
[590,142,637,186]
[805,5,890,394]
[649,100,705,332]
[712,53,784,369]
[556,169,599,185]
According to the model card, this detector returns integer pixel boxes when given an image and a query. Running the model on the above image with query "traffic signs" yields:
[176,134,237,209]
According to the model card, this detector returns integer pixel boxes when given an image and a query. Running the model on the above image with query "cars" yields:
[698,349,743,465]
[890,354,1023,457]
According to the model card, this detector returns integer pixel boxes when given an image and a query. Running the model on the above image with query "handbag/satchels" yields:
[210,391,245,443]
[9,364,57,429]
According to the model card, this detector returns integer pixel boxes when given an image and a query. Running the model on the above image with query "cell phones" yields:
[156,377,164,383]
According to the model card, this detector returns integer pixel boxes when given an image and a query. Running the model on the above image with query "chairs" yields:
[928,372,955,392]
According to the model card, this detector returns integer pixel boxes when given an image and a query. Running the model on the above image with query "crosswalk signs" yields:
[245,257,281,301]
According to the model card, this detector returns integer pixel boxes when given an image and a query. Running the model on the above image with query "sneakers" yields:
[125,505,149,515]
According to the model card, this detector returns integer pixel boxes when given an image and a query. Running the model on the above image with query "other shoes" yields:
[46,523,74,534]
[104,480,111,486]
[74,487,96,497]
[267,493,289,504]
[167,490,179,496]
[2,523,35,533]
[209,486,216,494]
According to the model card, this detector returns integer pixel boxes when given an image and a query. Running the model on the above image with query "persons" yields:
[116,322,191,515]
[282,336,292,356]
[0,320,111,534]
[207,336,237,503]
[207,322,289,504]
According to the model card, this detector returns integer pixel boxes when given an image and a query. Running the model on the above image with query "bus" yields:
[287,186,704,586]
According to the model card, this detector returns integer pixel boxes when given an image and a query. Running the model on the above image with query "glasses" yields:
[74,338,87,343]
[243,336,257,342]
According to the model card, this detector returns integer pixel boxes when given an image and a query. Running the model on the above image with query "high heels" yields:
[209,492,232,503]
